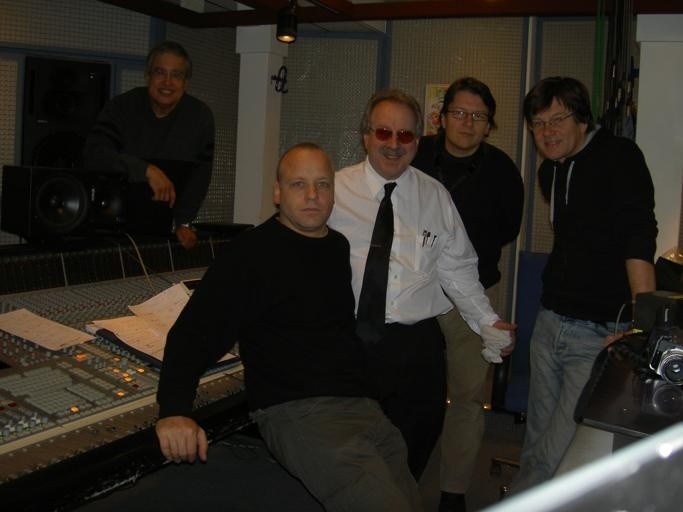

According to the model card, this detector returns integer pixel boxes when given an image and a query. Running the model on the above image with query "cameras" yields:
[642,324,683,386]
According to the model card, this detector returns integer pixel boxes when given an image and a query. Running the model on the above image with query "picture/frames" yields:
[423,83,450,138]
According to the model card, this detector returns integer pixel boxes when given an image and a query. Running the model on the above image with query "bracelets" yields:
[180,221,192,229]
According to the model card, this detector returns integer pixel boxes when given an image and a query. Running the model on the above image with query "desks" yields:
[573,335,680,454]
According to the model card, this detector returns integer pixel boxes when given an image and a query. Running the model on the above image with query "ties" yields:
[355,182,398,356]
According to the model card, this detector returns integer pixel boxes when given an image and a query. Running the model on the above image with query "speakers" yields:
[22,56,110,166]
[1,164,91,240]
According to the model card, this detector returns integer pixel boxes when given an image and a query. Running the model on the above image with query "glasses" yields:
[369,126,415,144]
[446,110,489,122]
[529,111,574,131]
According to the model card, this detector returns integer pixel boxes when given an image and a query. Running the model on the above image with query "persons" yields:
[324,86,517,483]
[498,76,661,498]
[407,76,525,510]
[154,142,423,511]
[87,41,217,248]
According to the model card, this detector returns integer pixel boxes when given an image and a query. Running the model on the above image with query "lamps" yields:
[274,0,301,44]
